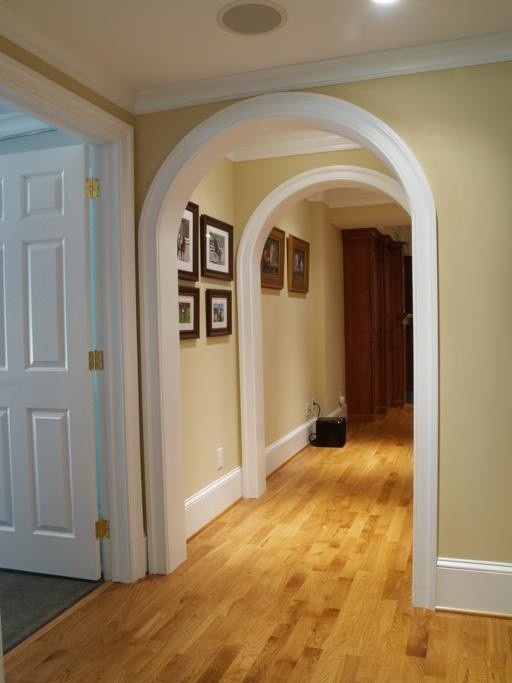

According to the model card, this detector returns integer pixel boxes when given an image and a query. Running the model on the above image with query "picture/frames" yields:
[176,200,234,339]
[261,225,311,293]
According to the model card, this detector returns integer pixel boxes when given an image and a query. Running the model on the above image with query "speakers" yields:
[316,416,346,447]
